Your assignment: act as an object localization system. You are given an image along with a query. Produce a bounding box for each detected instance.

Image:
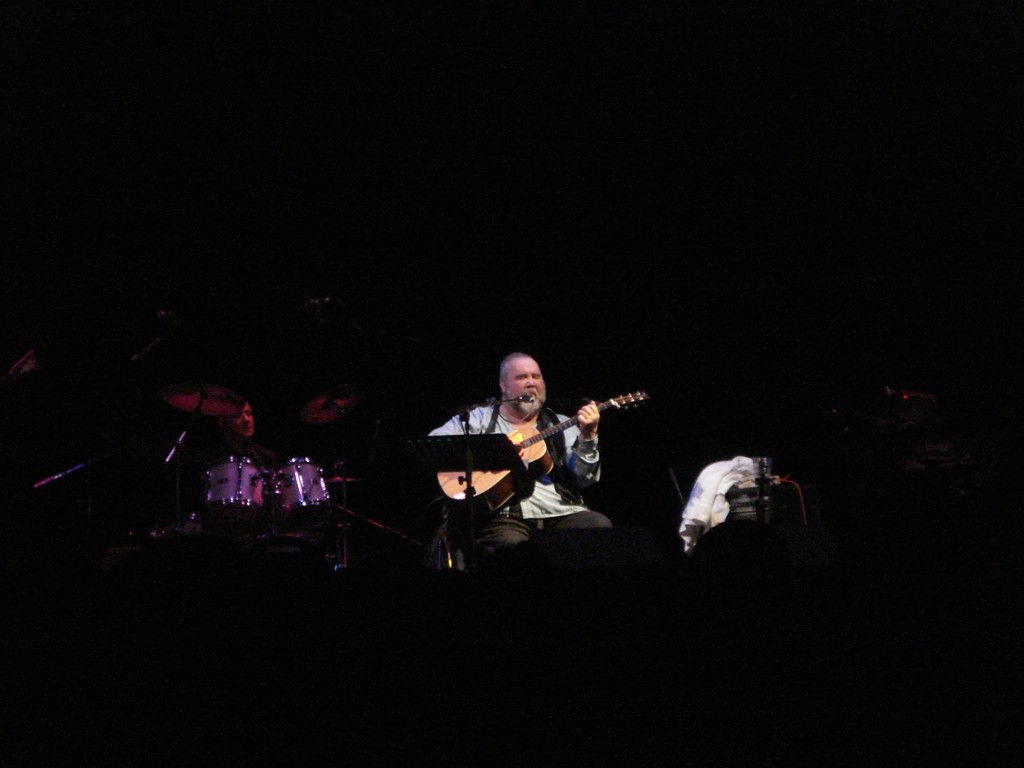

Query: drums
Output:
[180,515,204,536]
[196,453,262,531]
[266,457,332,526]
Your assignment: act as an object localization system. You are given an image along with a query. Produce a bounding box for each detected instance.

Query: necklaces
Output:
[498,405,531,423]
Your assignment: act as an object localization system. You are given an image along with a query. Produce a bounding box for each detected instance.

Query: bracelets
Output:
[572,435,599,454]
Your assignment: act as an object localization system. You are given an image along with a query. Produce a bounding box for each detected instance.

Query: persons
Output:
[677,454,774,552]
[427,353,610,544]
[200,400,276,469]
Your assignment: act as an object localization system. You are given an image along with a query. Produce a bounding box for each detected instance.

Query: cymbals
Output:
[300,382,357,425]
[324,475,362,485]
[162,384,245,418]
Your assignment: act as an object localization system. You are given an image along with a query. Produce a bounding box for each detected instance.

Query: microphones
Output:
[521,392,535,403]
[753,457,771,524]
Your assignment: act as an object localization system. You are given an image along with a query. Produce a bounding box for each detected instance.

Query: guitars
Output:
[437,390,652,515]
